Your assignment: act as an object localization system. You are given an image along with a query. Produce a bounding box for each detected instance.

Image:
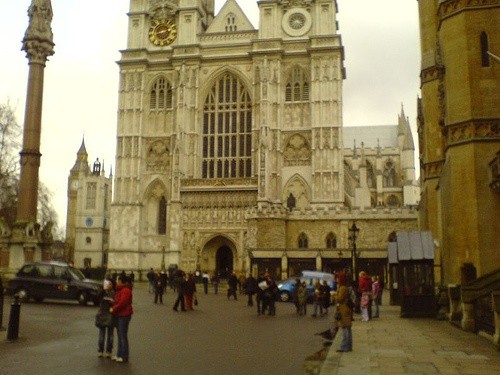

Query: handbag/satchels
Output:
[95,313,114,327]
[335,311,341,321]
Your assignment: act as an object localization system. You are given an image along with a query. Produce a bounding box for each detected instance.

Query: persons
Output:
[330,268,354,352]
[201,271,211,294]
[227,272,277,317]
[107,268,134,281]
[146,267,169,304]
[97,275,134,362]
[137,270,142,281]
[172,264,197,312]
[211,272,219,294]
[358,271,383,320]
[292,279,331,318]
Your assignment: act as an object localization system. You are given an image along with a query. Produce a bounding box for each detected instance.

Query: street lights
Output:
[348,221,361,284]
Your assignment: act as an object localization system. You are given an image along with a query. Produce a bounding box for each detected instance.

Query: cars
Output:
[3,262,103,307]
[275,270,340,303]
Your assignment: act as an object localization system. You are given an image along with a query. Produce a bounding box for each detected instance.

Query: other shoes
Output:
[105,352,111,358]
[115,357,124,362]
[99,352,104,357]
[173,308,178,311]
[111,355,116,360]
[336,348,350,352]
